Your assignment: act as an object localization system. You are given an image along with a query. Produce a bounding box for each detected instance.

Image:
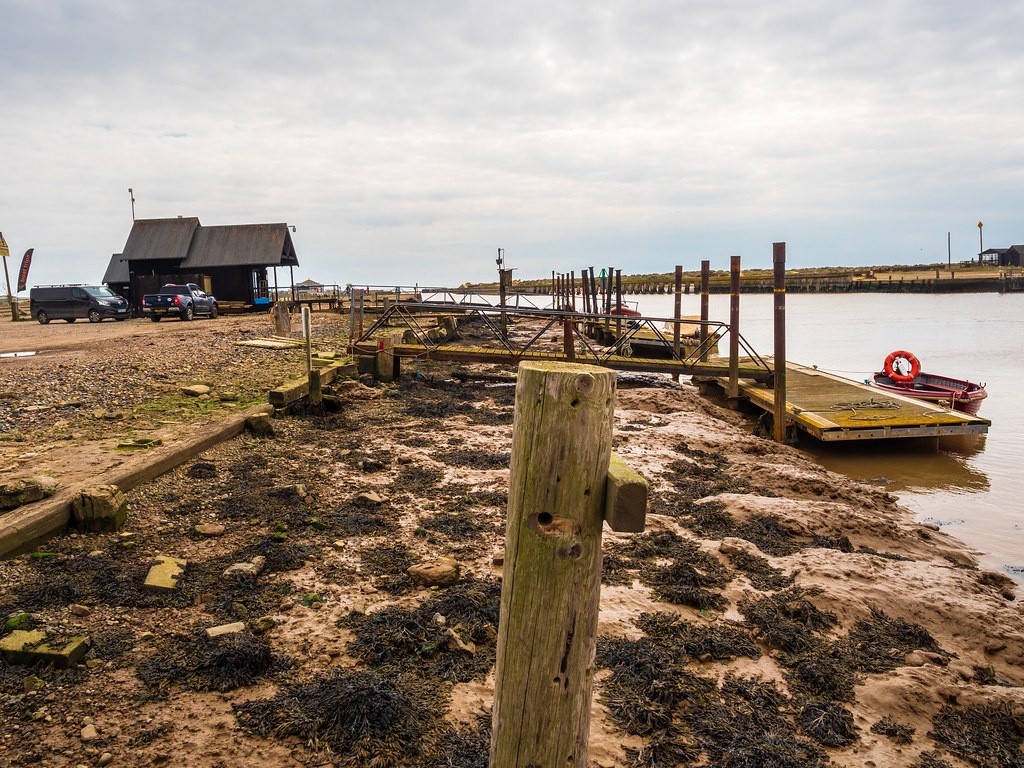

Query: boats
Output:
[601,301,641,320]
[663,313,720,349]
[865,368,988,417]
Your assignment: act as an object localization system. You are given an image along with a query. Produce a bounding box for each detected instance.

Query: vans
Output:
[30,282,130,323]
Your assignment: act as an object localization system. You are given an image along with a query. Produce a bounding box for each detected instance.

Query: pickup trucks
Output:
[143,283,219,322]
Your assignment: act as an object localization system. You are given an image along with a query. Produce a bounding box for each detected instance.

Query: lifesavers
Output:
[884,350,921,383]
